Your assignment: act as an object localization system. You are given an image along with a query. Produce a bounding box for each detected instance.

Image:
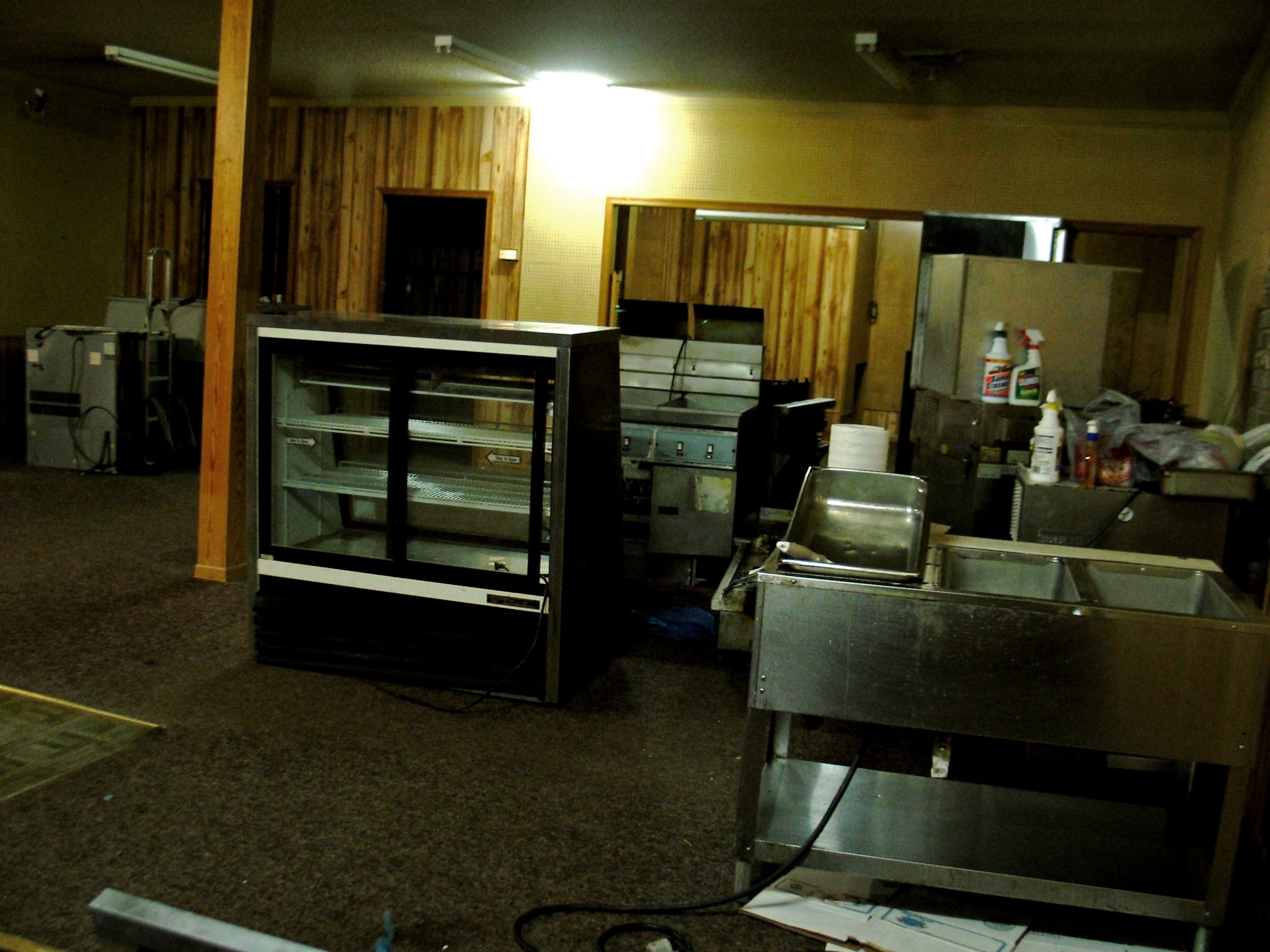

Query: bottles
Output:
[1031,389,1064,484]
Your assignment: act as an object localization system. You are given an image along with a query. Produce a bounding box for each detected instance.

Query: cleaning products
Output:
[980,320,1015,403]
[1028,386,1065,484]
[1007,327,1045,407]
[1081,418,1102,489]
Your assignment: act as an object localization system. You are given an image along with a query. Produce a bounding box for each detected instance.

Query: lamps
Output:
[434,35,531,85]
[854,33,913,92]
[105,45,218,86]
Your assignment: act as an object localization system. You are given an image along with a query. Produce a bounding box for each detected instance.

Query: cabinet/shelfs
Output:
[242,309,622,708]
